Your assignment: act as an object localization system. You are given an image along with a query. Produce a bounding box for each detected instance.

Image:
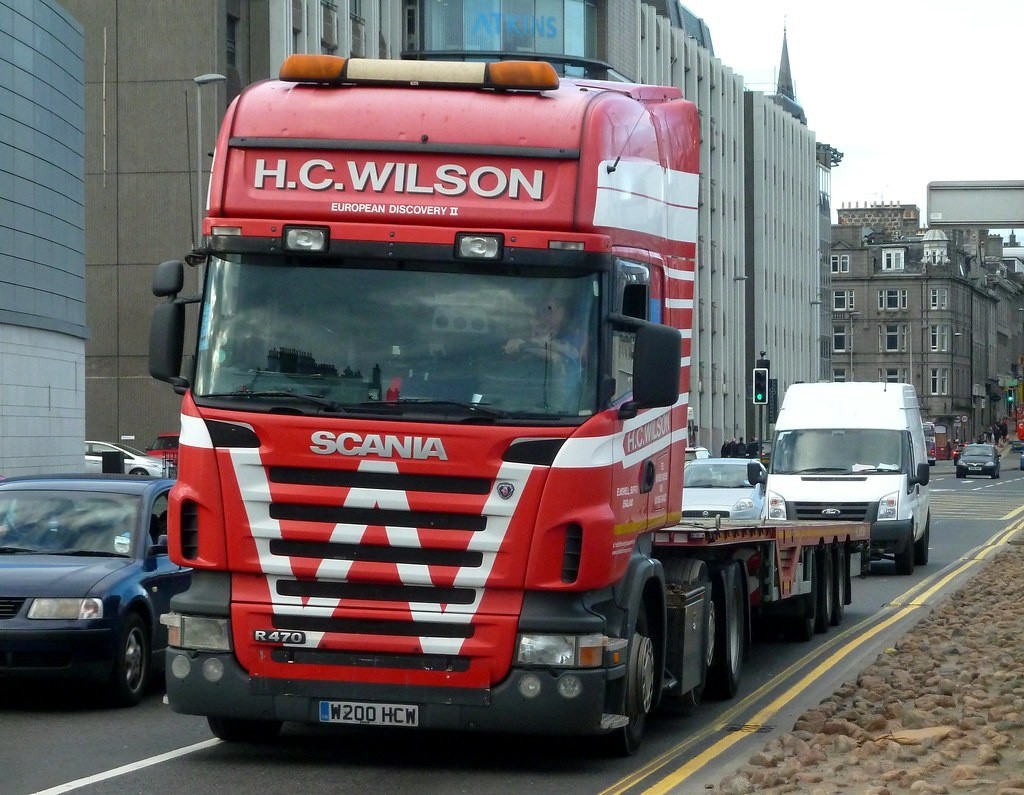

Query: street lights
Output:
[186,68,225,293]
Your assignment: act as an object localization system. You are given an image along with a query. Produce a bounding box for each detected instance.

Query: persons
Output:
[978,417,1008,445]
[504,296,587,370]
[946,439,952,457]
[720,438,759,459]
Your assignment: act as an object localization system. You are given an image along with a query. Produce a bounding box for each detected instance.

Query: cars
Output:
[86,439,176,477]
[955,444,1002,479]
[0,474,195,702]
[1020,448,1024,471]
[682,456,768,520]
[145,430,180,466]
[953,442,970,466]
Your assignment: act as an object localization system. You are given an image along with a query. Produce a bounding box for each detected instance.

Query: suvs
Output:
[684,445,711,463]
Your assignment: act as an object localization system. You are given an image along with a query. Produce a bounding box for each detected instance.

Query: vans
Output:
[746,379,932,577]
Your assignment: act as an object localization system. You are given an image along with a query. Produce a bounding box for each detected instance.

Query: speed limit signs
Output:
[960,414,970,424]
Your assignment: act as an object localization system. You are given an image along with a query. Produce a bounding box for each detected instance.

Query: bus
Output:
[922,421,937,467]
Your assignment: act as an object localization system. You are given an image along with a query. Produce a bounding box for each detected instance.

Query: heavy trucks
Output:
[138,44,876,757]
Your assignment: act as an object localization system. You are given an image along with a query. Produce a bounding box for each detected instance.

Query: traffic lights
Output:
[750,367,769,409]
[1007,388,1015,408]
